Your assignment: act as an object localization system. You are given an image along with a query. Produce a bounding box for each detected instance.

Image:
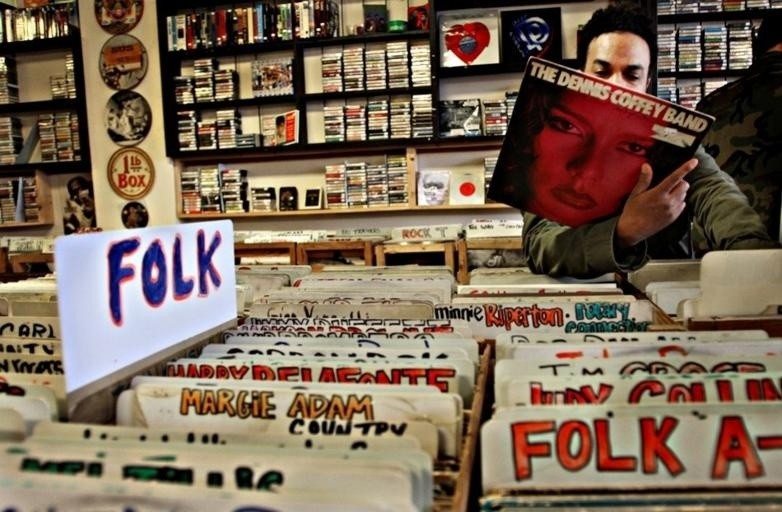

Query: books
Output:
[167,6,297,213]
[657,1,781,109]
[439,90,519,190]
[294,0,434,207]
[1,262,782,512]
[1,5,81,254]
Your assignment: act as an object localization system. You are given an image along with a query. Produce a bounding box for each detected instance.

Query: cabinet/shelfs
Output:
[1,1,777,284]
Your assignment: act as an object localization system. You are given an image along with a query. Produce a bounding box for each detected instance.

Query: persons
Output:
[692,14,782,257]
[522,6,773,279]
